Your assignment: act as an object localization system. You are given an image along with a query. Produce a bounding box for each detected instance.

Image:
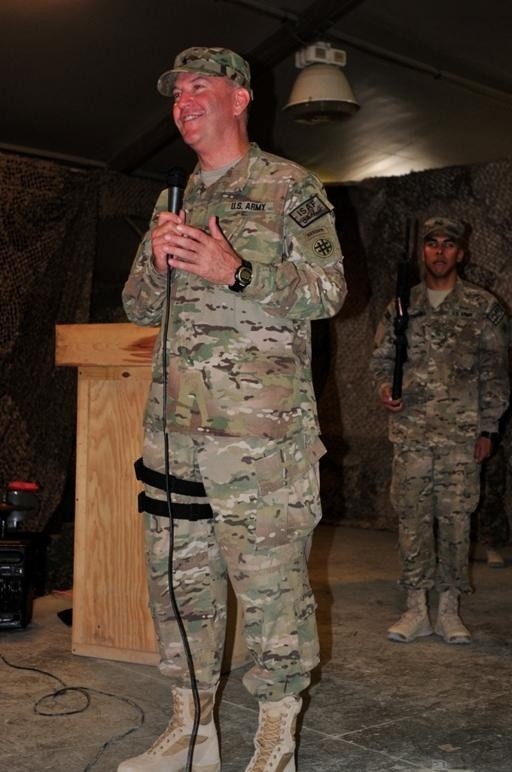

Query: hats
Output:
[422,215,467,242]
[156,46,252,98]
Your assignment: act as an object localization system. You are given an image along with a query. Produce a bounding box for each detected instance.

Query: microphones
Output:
[159,160,189,244]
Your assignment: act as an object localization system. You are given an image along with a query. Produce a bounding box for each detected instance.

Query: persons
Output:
[115,43,350,771]
[370,216,506,646]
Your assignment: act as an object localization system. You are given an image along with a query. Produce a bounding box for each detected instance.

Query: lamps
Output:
[279,44,364,127]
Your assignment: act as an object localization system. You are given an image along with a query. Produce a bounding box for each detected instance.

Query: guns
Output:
[392,219,421,401]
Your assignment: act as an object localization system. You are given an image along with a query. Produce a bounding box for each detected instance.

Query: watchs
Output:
[228,259,252,293]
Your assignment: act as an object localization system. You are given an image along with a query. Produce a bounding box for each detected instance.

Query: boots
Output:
[240,694,305,772]
[386,588,435,644]
[434,589,473,645]
[118,679,221,772]
